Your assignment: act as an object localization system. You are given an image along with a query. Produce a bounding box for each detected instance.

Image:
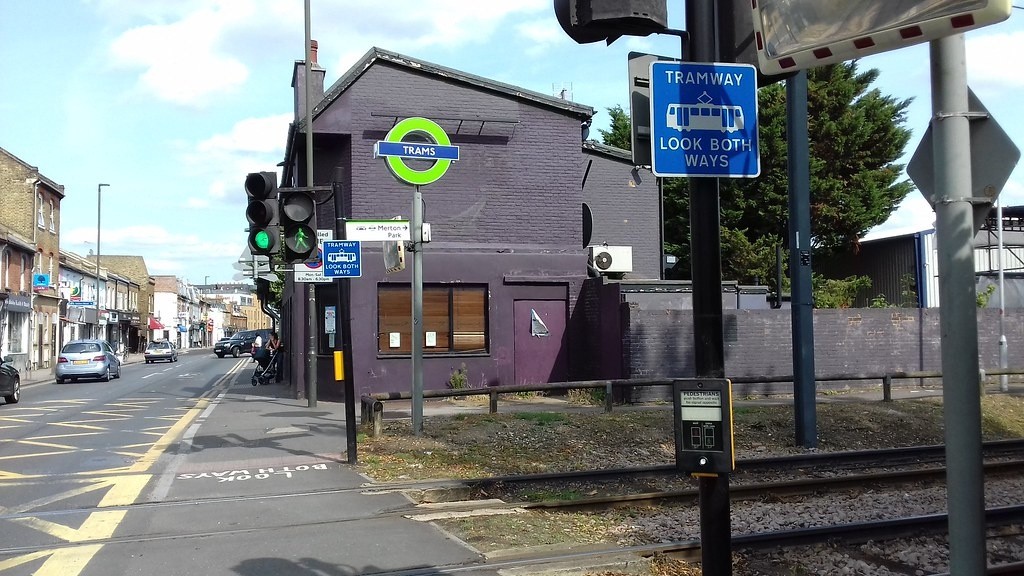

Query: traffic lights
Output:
[244,171,282,256]
[200,326,205,332]
[278,189,320,264]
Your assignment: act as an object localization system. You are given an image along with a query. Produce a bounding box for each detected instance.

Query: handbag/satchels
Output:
[251,344,256,355]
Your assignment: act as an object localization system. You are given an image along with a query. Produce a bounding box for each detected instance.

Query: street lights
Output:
[95,182,110,343]
[204,275,210,301]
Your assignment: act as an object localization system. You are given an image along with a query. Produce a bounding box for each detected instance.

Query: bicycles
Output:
[122,346,132,363]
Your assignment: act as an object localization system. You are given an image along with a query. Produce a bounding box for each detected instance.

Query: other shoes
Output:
[252,361,255,363]
[275,381,280,383]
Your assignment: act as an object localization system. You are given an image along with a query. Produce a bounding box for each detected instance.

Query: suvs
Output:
[214,328,273,358]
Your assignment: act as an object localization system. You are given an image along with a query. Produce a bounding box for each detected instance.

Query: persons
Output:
[252,332,262,363]
[266,332,284,379]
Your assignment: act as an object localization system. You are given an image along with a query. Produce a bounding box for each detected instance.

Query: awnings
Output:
[150,317,165,329]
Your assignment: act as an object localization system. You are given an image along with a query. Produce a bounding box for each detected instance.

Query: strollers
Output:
[251,347,279,387]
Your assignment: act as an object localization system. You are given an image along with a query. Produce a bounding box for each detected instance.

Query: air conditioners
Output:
[588,244,634,272]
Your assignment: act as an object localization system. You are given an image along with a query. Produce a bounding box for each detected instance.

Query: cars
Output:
[0,356,20,404]
[144,338,178,364]
[54,338,122,384]
